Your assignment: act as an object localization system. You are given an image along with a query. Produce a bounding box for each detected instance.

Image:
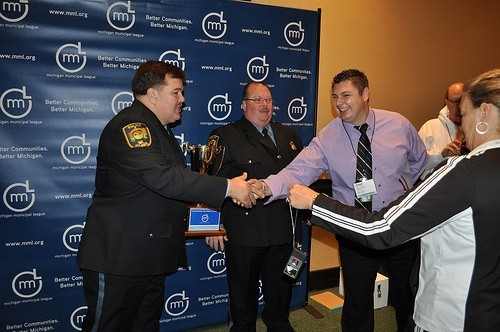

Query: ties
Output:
[261,128,276,148]
[353,123,372,213]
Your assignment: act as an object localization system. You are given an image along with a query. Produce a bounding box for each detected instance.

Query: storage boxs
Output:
[338,267,389,310]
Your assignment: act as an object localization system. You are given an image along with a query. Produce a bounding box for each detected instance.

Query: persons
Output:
[76,60,264,332]
[417,81,464,181]
[206,81,304,332]
[232,68,427,331]
[289,68,500,332]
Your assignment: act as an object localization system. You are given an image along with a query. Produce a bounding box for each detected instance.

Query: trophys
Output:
[179,134,226,237]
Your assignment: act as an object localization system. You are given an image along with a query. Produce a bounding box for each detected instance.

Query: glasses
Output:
[448,99,460,104]
[245,97,273,104]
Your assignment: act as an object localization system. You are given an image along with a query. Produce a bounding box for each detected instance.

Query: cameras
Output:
[282,247,308,280]
